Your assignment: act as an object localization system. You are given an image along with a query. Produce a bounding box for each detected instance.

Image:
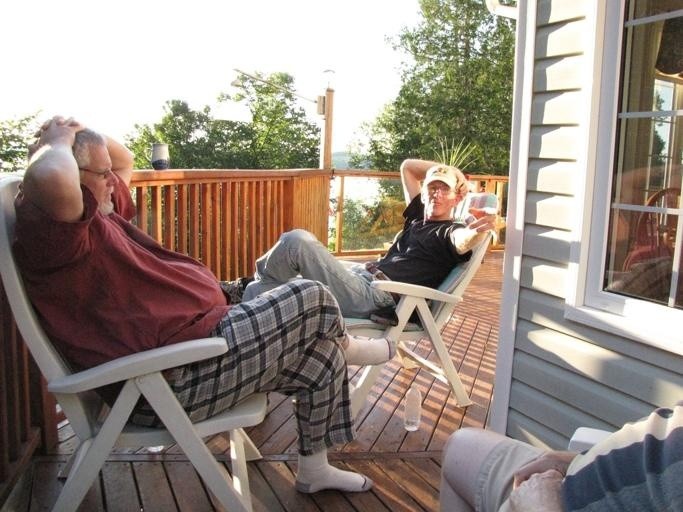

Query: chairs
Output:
[344,192,499,420]
[0,177,266,512]
[568,426,613,454]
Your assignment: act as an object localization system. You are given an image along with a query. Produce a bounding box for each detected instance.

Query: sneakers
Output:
[219,277,245,304]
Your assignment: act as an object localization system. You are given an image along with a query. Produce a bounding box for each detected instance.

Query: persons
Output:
[10,113,400,495]
[437,398,683,512]
[214,158,498,321]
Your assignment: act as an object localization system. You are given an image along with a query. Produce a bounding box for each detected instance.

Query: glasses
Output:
[427,184,455,196]
[78,165,112,181]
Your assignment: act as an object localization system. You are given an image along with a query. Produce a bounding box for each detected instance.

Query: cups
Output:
[145,143,171,170]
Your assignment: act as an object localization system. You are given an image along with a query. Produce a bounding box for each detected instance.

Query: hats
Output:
[423,165,458,191]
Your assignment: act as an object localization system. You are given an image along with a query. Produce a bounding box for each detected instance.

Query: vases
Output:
[152,144,169,168]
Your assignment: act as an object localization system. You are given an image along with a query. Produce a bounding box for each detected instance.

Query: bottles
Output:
[403,384,422,431]
